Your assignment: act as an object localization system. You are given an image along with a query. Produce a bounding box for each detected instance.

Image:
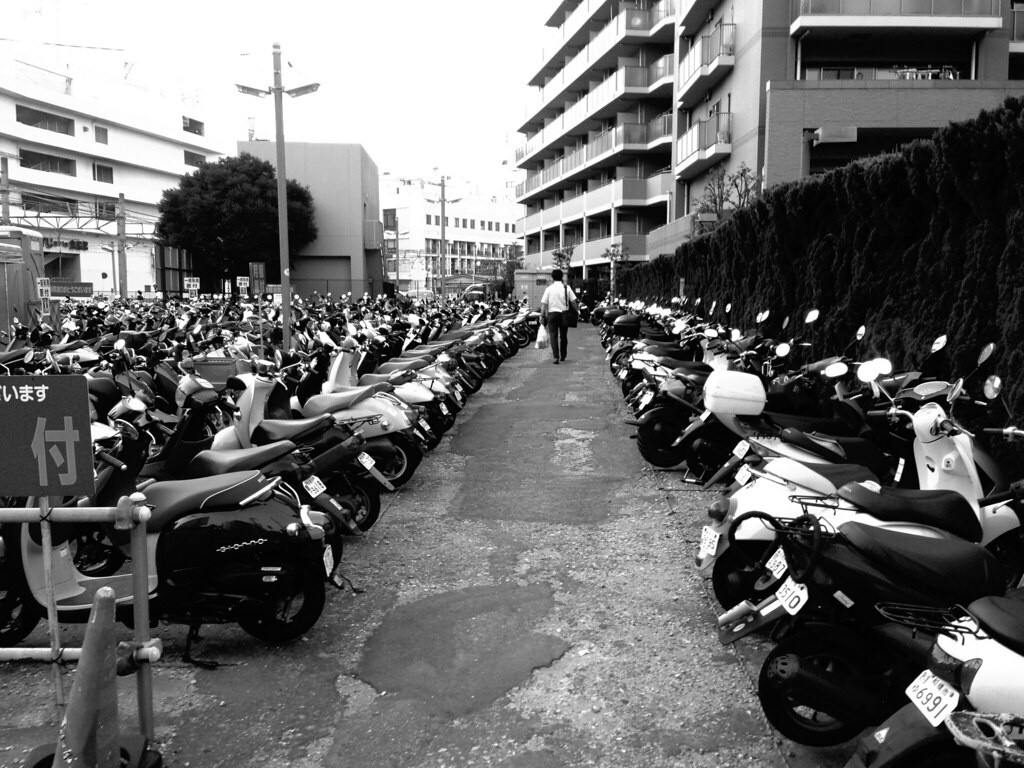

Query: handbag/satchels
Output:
[535,324,550,349]
[563,309,577,327]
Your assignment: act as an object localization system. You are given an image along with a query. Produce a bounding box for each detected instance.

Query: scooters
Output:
[574,286,1024,611]
[845,595,1024,768]
[0,417,333,660]
[716,513,1024,748]
[755,376,1024,641]
[0,292,548,588]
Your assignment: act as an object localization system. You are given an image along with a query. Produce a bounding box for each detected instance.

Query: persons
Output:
[539,269,579,364]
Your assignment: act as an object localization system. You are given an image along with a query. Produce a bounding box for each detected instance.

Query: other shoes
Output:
[553,358,559,364]
[561,355,566,361]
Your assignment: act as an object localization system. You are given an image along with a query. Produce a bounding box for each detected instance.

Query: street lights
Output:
[386,231,410,294]
[235,80,320,353]
[426,197,462,308]
